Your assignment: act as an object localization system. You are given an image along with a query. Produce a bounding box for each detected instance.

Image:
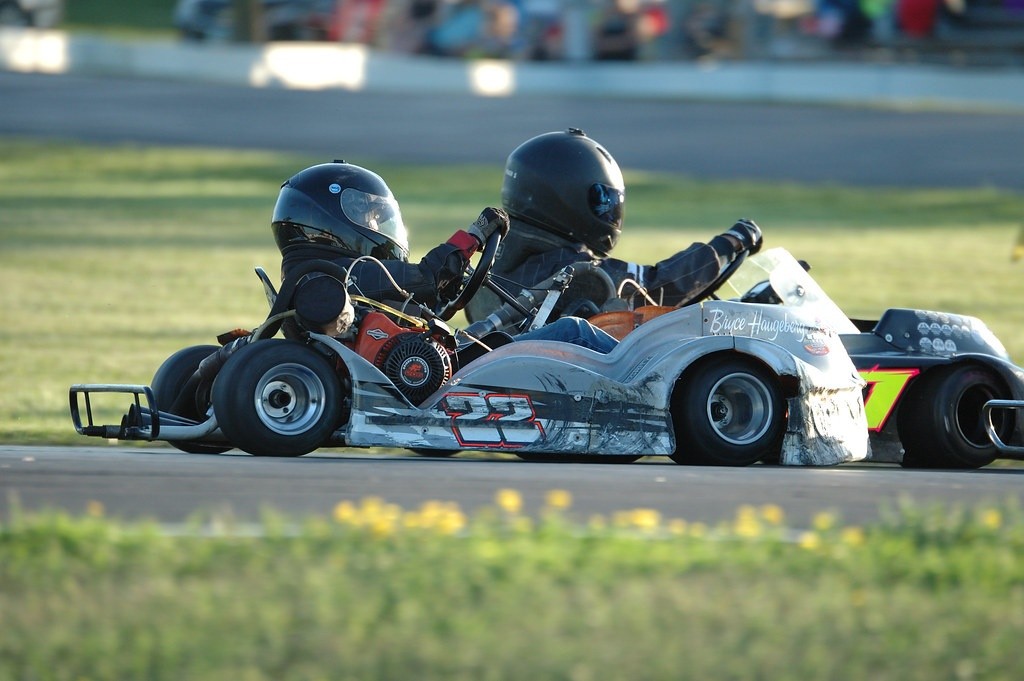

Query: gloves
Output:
[721,218,763,257]
[467,207,510,252]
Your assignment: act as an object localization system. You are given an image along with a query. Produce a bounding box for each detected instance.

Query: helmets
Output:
[271,160,410,281]
[502,127,625,255]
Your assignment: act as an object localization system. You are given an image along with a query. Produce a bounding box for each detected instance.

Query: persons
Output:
[272,159,621,357]
[464,126,764,335]
[0,0,1024,60]
